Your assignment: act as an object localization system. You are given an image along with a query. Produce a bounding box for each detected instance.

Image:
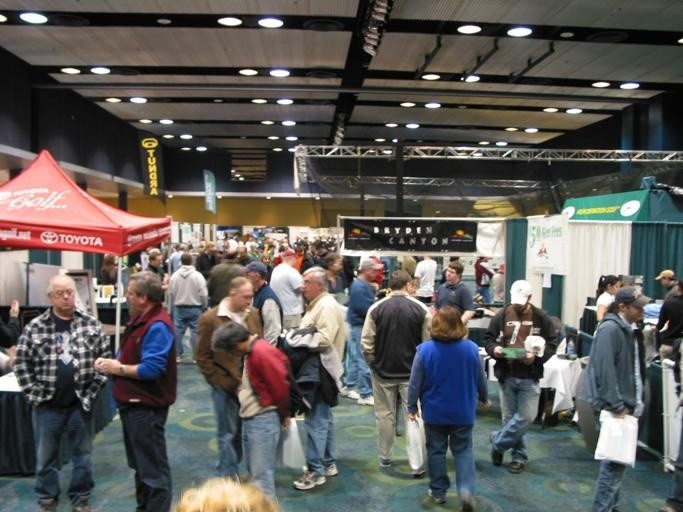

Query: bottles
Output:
[567,338,574,354]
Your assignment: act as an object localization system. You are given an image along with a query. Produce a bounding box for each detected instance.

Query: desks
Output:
[0,371,112,475]
[96,297,131,326]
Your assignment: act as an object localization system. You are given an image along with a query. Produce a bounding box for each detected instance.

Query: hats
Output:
[616,286,651,307]
[655,270,674,280]
[511,280,533,305]
[240,261,267,272]
[362,259,383,269]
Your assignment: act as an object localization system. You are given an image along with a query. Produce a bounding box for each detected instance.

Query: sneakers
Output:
[428,490,445,504]
[340,387,359,399]
[294,471,326,489]
[302,463,338,476]
[379,456,392,466]
[358,396,374,405]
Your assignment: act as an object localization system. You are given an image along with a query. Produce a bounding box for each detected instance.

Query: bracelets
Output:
[119,365,125,374]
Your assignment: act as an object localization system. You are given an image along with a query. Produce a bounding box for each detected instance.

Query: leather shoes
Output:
[509,461,524,473]
[490,431,503,466]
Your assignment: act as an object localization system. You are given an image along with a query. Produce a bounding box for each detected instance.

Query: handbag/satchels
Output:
[594,410,639,467]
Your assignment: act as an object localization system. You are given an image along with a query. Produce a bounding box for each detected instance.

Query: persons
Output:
[585,274,652,512]
[485,279,576,473]
[346,255,505,477]
[194,253,346,489]
[95,271,178,512]
[175,476,280,512]
[654,269,683,512]
[1,299,20,350]
[15,275,110,512]
[99,253,120,287]
[407,306,490,512]
[133,235,345,365]
